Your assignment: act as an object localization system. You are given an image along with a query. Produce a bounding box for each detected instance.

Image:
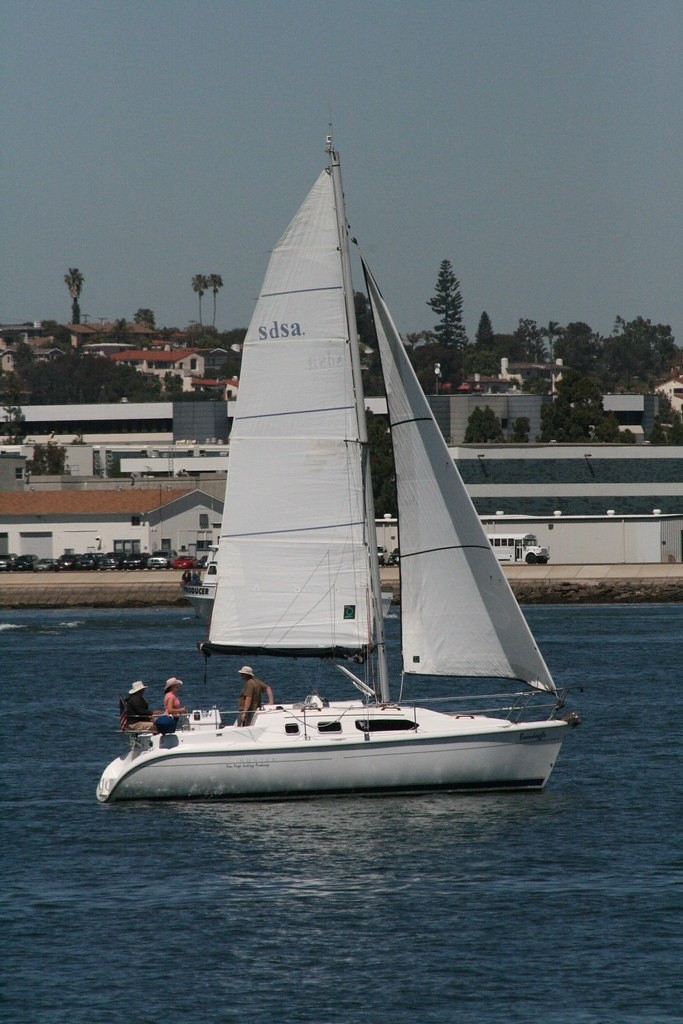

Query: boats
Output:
[179,546,218,620]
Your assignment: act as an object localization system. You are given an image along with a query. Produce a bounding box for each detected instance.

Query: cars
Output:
[376,545,386,567]
[33,558,58,572]
[198,555,209,567]
[172,554,200,570]
[387,547,400,566]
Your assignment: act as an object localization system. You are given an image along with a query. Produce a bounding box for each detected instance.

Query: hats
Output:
[165,677,183,690]
[238,666,254,676]
[128,681,148,694]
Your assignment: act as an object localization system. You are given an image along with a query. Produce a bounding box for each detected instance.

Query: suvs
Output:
[0,553,16,571]
[52,554,82,572]
[13,554,39,571]
[123,552,151,571]
[76,552,105,571]
[147,549,178,570]
[98,552,127,571]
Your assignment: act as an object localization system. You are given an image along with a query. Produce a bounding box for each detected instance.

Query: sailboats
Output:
[96,129,582,802]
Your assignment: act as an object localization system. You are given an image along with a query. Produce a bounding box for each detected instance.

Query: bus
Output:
[483,533,551,564]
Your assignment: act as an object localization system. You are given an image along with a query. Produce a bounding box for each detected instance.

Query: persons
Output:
[236,666,274,727]
[127,680,162,735]
[163,677,187,732]
[181,569,203,585]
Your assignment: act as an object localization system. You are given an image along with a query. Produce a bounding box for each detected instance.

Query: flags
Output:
[119,693,127,732]
